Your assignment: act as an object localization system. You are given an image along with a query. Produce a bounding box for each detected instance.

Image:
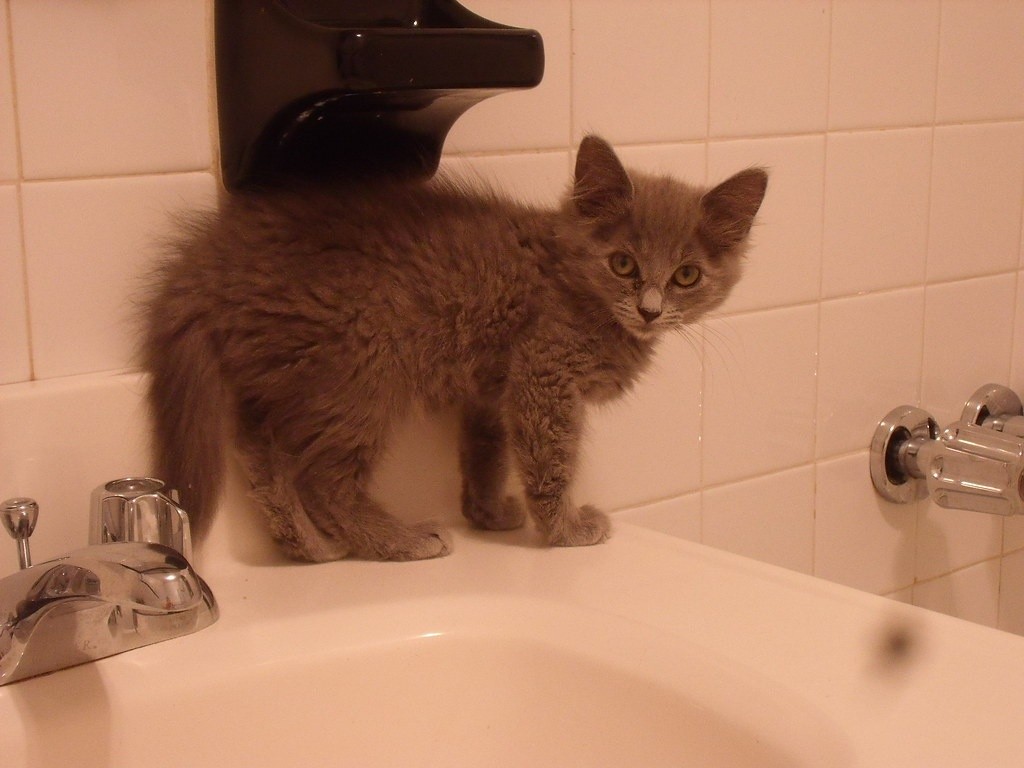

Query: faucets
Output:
[37,542,202,613]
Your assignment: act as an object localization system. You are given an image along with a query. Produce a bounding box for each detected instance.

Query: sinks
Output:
[1,518,1022,768]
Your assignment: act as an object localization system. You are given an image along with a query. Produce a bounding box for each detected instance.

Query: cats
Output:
[119,132,772,563]
